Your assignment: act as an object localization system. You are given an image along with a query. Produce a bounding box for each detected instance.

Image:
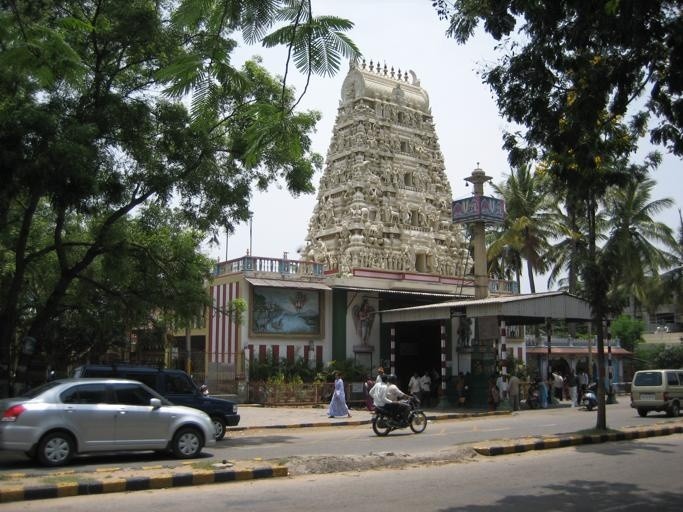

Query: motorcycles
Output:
[527,381,555,410]
[581,381,599,412]
[367,393,428,437]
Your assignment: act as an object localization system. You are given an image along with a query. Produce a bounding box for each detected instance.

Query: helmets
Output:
[389,374,398,383]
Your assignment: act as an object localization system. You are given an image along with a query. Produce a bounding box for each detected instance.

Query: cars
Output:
[1,376,219,470]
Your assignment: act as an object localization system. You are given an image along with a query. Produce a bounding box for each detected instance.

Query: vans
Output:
[63,358,241,441]
[628,368,683,418]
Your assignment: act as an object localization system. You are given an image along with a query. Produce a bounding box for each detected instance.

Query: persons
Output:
[359,303,369,346]
[463,318,472,347]
[326,372,351,418]
[365,367,589,430]
